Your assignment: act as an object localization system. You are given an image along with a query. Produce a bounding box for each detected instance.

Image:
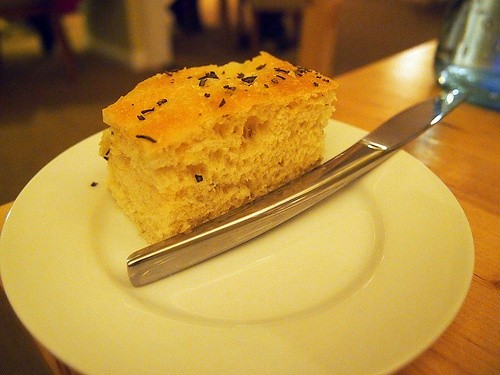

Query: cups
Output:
[433,1,500,110]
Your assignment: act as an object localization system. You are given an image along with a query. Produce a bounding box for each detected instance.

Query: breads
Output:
[102,50,337,245]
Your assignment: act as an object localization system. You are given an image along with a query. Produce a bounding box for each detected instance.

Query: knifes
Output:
[126,90,473,288]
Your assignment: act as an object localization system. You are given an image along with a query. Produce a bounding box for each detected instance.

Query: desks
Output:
[0,37,500,375]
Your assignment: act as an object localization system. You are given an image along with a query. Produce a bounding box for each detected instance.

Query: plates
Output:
[0,118,476,375]
